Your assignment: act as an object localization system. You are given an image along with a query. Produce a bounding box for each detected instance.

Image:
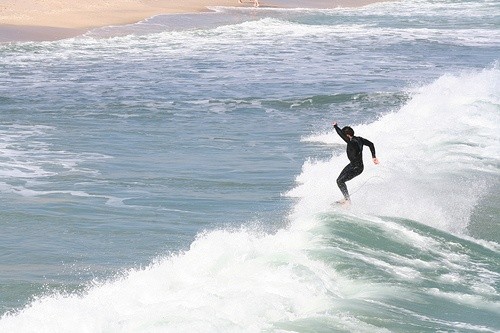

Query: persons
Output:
[332,120,380,205]
